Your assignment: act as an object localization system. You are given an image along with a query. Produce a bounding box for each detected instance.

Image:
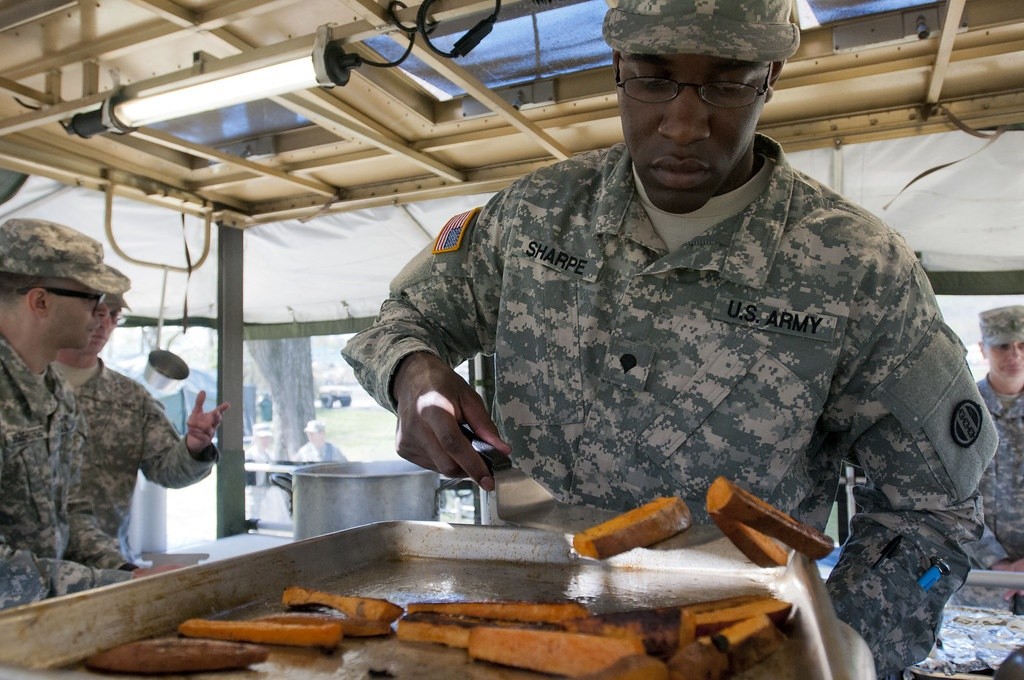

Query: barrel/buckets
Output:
[269,459,465,540]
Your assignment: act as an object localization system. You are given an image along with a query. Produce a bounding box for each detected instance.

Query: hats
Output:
[253,424,273,438]
[0,218,131,295]
[304,420,327,434]
[101,294,131,312]
[603,0,803,63]
[980,304,1024,348]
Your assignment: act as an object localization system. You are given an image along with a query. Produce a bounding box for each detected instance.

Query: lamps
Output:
[66,28,362,138]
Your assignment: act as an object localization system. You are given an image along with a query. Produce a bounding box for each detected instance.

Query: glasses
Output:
[16,284,107,316]
[615,49,775,109]
[97,305,127,326]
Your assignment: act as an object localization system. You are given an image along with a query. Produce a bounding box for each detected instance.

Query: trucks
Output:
[313,374,353,408]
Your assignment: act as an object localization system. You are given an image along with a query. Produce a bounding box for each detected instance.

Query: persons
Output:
[51,265,231,563]
[292,420,348,462]
[948,304,1024,611]
[0,217,182,608]
[245,422,274,519]
[339,0,999,680]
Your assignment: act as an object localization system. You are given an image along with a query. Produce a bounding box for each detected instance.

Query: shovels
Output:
[458,423,627,535]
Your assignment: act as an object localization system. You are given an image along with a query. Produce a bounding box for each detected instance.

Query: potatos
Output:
[83,473,837,680]
